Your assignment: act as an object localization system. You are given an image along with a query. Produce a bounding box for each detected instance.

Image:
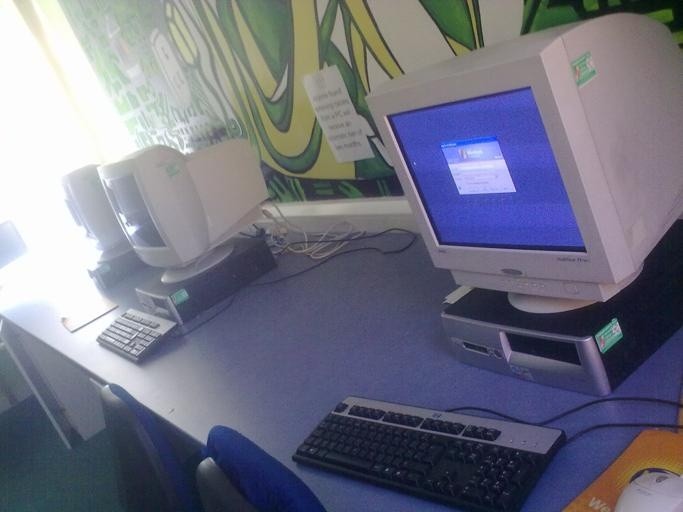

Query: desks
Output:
[0,233,683,511]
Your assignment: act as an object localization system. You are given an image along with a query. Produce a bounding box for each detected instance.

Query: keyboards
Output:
[96,308,178,362]
[292,395,567,512]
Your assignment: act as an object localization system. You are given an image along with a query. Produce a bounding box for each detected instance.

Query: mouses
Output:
[614,466,683,512]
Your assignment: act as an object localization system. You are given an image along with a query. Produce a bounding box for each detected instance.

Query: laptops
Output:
[365,12,682,315]
[96,137,270,283]
[52,164,136,261]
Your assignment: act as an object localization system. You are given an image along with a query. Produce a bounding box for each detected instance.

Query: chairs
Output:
[100,382,332,512]
